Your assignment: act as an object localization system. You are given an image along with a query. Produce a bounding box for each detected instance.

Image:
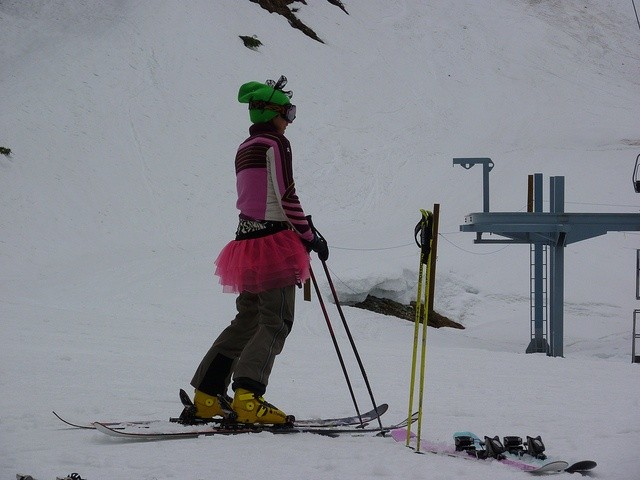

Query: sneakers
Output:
[230,388,287,424]
[194,388,234,419]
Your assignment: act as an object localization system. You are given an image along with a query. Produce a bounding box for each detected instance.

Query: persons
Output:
[190,75,329,425]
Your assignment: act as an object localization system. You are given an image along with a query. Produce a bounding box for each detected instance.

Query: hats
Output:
[238,81,290,124]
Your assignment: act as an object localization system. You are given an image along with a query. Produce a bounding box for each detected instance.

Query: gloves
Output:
[309,234,329,262]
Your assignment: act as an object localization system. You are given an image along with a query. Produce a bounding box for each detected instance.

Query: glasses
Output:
[249,99,296,123]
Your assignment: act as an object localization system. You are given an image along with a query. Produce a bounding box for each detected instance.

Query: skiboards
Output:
[390,428,596,473]
[52,404,419,437]
